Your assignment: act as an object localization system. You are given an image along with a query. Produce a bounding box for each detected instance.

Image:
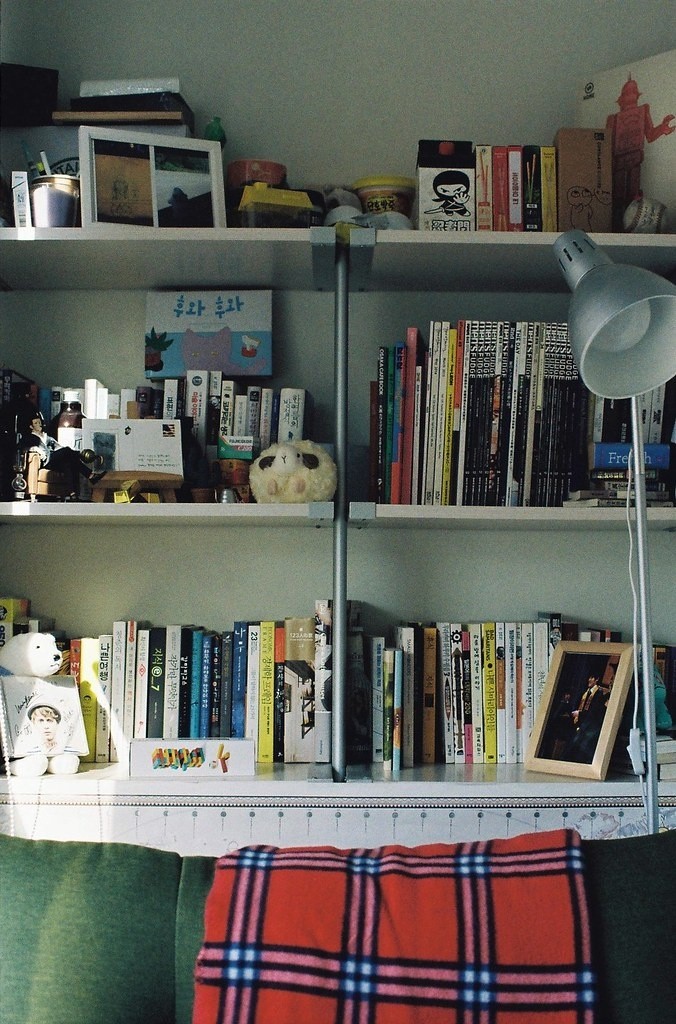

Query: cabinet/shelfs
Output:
[0,232,676,799]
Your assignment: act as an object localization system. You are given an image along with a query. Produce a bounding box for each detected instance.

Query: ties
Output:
[582,691,592,710]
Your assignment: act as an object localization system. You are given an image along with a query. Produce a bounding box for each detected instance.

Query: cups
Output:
[352,173,418,219]
[30,174,79,228]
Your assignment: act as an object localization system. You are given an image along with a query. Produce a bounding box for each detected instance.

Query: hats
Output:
[28,702,60,724]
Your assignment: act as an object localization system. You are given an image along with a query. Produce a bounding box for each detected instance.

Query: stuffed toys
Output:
[246,439,338,503]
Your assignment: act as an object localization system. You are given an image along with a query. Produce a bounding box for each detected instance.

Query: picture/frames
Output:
[521,639,639,783]
[78,124,227,229]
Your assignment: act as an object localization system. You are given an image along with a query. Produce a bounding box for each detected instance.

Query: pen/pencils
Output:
[19,139,40,178]
[35,161,47,176]
[40,149,52,177]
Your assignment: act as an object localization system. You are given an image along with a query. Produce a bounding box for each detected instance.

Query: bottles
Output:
[48,389,91,500]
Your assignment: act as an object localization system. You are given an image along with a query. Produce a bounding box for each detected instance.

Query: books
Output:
[0,367,309,504]
[142,284,274,382]
[368,313,676,507]
[410,125,616,234]
[352,607,676,781]
[44,107,187,128]
[0,595,333,775]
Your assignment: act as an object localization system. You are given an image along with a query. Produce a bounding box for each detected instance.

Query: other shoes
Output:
[70,494,83,503]
[90,471,108,485]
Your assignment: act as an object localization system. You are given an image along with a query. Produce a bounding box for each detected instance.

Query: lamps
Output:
[549,233,676,837]
